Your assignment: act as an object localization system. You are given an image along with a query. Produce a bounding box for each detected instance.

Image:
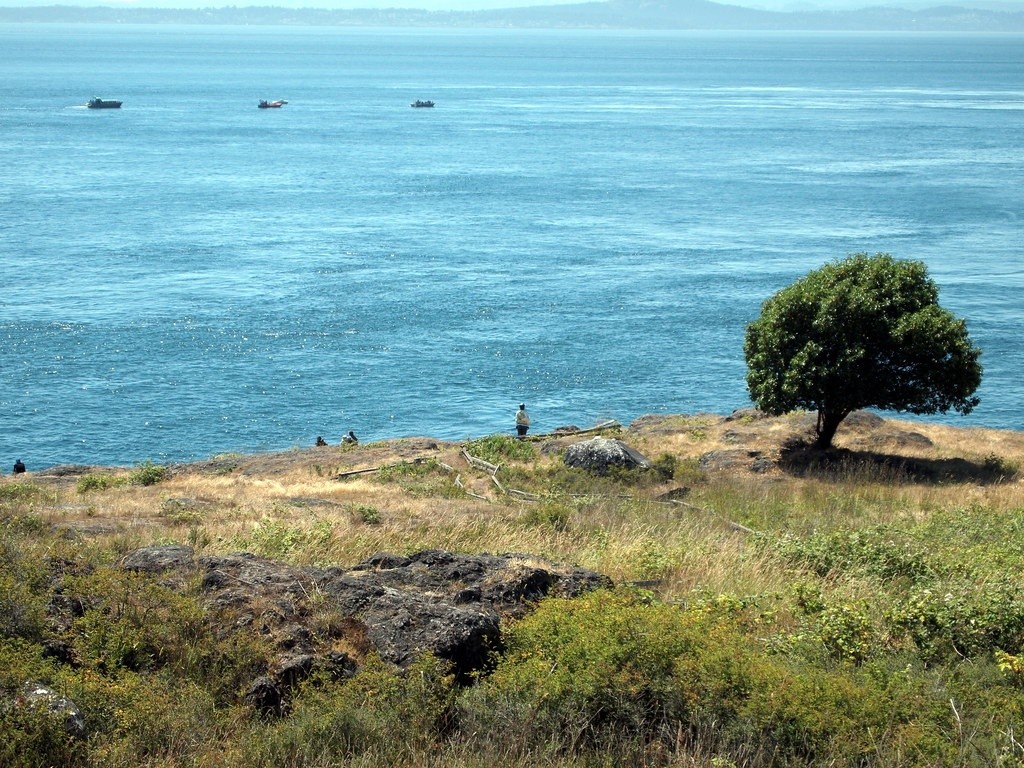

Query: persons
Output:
[341,435,348,446]
[316,436,327,446]
[347,431,358,446]
[515,403,529,436]
[14,458,26,474]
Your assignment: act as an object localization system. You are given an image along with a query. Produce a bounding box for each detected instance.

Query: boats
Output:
[410,99,435,107]
[258,98,282,108]
[85,96,123,108]
[273,99,288,104]
